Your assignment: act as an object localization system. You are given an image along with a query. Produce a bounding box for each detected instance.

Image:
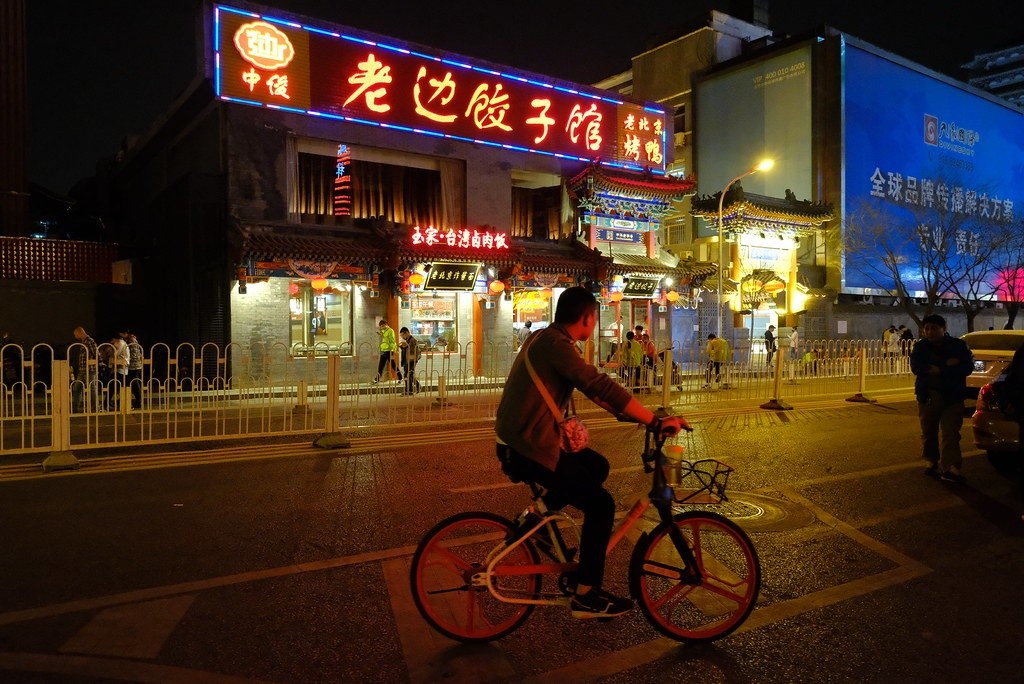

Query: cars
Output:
[971,364,1024,482]
[956,329,1024,400]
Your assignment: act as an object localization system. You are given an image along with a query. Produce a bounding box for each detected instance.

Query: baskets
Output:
[666,459,734,504]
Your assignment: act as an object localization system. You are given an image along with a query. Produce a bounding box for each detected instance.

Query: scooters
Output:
[625,346,683,393]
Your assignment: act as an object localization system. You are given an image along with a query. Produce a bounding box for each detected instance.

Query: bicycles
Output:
[409,406,762,646]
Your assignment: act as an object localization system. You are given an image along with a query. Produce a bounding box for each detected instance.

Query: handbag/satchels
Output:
[558,415,588,453]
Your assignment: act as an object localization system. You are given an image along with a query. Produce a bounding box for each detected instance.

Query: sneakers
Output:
[571,588,633,618]
[513,510,553,546]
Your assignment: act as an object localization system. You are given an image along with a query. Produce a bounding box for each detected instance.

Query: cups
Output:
[665,445,683,487]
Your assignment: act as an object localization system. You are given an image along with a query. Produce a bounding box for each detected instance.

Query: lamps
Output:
[422,263,432,272]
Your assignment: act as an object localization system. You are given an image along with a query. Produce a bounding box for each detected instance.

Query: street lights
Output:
[717,158,776,338]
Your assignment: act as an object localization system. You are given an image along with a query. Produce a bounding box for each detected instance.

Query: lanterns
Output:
[666,290,679,304]
[310,278,328,293]
[408,273,424,288]
[611,292,622,302]
[743,278,784,297]
[490,280,504,292]
[513,288,552,311]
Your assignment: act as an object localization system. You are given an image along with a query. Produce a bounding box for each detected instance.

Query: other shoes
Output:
[941,471,955,482]
[401,392,413,396]
[924,462,938,475]
[398,379,403,383]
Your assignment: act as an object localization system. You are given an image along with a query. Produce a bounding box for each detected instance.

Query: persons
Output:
[702,334,728,388]
[438,336,446,346]
[319,311,325,329]
[989,327,994,330]
[882,324,913,365]
[998,343,1024,519]
[911,314,973,482]
[74,326,141,412]
[517,321,532,343]
[764,325,862,377]
[495,287,689,620]
[370,320,422,397]
[607,316,657,394]
